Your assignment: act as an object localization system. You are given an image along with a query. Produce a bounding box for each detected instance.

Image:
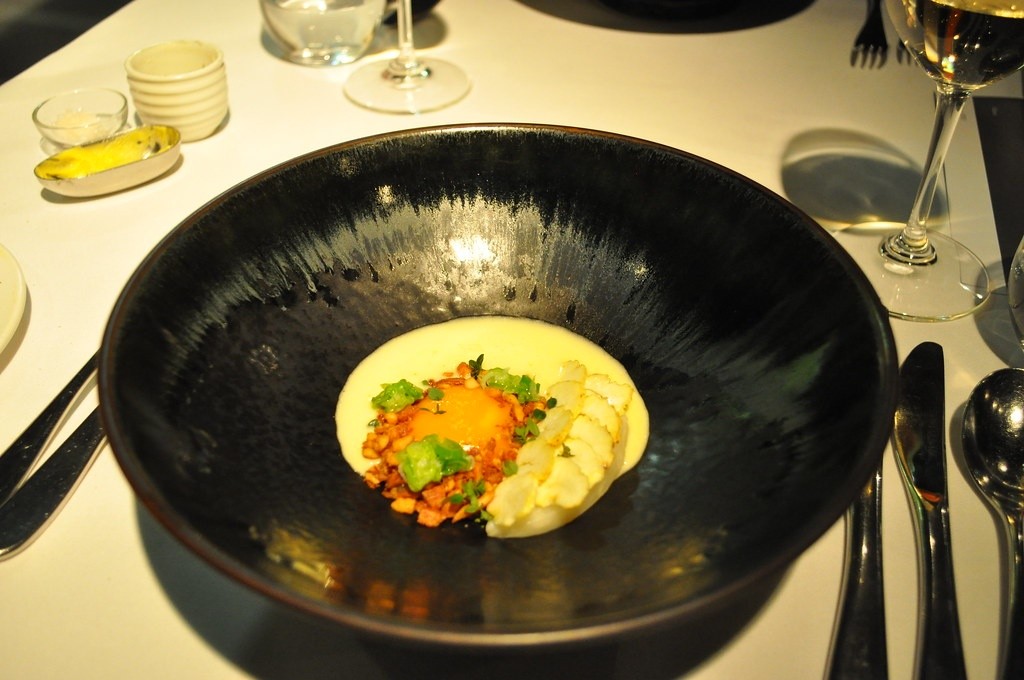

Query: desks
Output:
[0,0,1024,680]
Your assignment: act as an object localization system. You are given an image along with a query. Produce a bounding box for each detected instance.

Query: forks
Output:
[896,39,919,66]
[850,0,888,68]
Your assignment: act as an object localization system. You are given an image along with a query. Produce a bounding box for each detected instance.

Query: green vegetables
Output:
[368,351,559,523]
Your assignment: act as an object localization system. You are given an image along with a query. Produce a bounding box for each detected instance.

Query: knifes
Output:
[889,341,968,680]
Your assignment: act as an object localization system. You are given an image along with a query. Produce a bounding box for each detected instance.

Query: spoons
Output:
[960,368,1024,680]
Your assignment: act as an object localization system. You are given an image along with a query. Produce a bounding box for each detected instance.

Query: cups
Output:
[124,40,229,142]
[259,0,386,66]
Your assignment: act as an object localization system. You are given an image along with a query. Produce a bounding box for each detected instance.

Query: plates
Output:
[0,245,27,353]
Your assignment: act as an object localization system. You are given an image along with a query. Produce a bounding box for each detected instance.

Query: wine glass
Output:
[833,0,1024,324]
[342,0,471,115]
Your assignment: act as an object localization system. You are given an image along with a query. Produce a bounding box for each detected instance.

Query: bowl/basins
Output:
[31,87,128,151]
[34,124,183,198]
[96,122,902,654]
